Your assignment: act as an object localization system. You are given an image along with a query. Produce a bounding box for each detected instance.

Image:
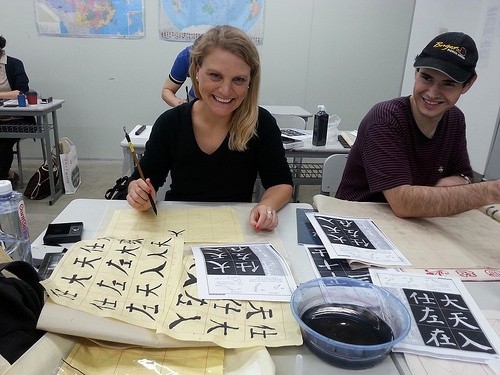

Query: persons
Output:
[0,36,38,179]
[127,24,295,233]
[334,32,500,218]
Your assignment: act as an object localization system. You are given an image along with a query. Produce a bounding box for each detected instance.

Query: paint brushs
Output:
[123,126,158,215]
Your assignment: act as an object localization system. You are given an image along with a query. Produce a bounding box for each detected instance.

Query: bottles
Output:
[0,179,34,268]
[312,104,329,146]
[18,92,26,107]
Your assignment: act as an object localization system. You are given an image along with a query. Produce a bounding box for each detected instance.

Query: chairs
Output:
[14,90,46,189]
[322,154,351,201]
[276,116,306,202]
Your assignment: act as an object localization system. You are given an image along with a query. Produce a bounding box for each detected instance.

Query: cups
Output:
[26,90,38,105]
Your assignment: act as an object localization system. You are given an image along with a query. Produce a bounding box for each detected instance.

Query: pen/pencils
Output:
[186,86,189,102]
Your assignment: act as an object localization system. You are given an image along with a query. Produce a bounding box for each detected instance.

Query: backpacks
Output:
[0,261,49,365]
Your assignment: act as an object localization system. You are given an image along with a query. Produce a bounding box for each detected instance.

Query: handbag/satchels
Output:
[24,137,80,200]
[105,176,131,200]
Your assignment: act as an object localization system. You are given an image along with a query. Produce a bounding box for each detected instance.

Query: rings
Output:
[268,211,273,214]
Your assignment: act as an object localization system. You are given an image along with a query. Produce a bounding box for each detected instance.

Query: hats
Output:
[413,32,478,83]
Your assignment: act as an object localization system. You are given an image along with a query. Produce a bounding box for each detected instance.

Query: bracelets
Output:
[460,173,470,182]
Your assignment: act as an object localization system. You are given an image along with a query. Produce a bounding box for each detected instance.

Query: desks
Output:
[121,126,358,203]
[0,99,64,206]
[30,199,500,375]
[260,105,313,130]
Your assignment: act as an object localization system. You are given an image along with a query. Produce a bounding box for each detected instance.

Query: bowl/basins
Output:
[290,276,411,371]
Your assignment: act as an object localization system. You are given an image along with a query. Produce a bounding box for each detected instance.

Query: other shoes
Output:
[6,172,19,190]
